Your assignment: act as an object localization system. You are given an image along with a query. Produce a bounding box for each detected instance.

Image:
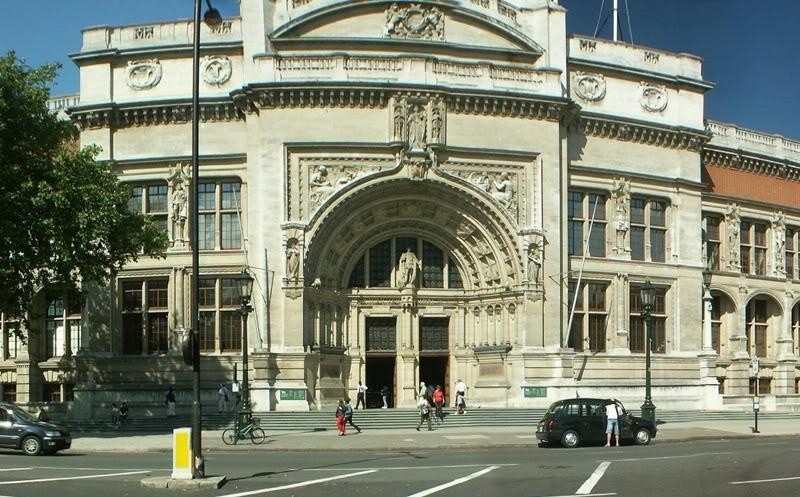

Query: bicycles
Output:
[222,411,266,446]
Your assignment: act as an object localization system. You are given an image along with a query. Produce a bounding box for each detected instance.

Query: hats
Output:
[436,385,441,389]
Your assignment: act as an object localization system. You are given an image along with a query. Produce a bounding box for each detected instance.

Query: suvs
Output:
[534,396,657,447]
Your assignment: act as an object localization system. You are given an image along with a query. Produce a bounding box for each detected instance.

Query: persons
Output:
[335,399,347,435]
[416,378,468,432]
[400,248,419,284]
[605,399,620,447]
[286,243,299,279]
[118,401,128,428]
[216,383,229,412]
[309,166,330,192]
[614,197,629,251]
[492,172,513,209]
[165,388,176,416]
[353,381,368,410]
[170,184,188,221]
[725,219,739,267]
[773,227,788,273]
[381,385,388,409]
[408,106,426,153]
[111,403,119,424]
[528,248,541,282]
[344,399,361,432]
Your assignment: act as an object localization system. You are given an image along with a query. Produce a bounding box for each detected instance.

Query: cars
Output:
[0,400,70,455]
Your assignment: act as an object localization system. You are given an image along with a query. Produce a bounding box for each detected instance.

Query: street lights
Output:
[640,277,656,422]
[186,0,220,480]
[698,268,718,386]
[237,262,256,437]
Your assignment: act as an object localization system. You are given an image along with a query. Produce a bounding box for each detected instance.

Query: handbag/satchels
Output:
[225,394,228,402]
